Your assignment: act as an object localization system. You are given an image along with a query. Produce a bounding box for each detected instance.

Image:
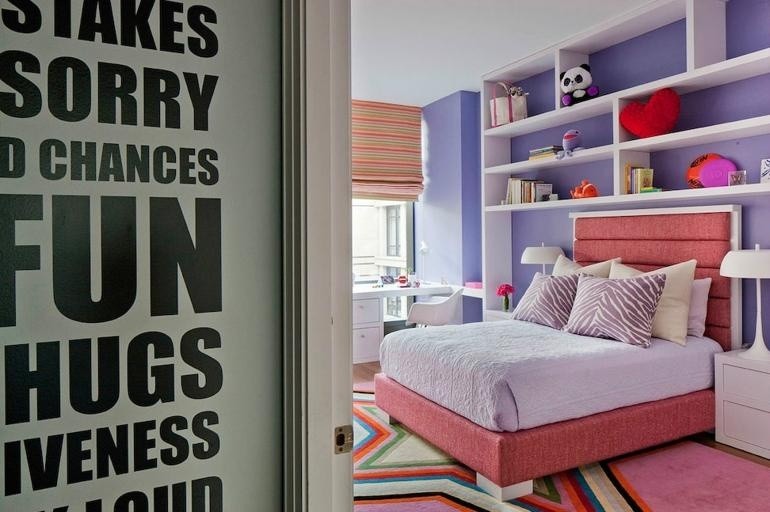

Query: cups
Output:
[408,271,416,284]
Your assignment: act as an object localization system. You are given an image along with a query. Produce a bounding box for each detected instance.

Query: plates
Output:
[686,152,722,189]
[701,158,737,189]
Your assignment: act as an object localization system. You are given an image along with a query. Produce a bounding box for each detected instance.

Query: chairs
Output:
[406,287,465,328]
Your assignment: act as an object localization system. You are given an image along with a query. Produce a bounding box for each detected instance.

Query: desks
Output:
[352,278,453,365]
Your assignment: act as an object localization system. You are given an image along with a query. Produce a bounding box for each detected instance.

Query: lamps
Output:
[519,240,566,276]
[719,243,770,361]
[417,239,431,285]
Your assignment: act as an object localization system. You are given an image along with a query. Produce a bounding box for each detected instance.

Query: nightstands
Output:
[713,348,770,461]
[486,308,514,321]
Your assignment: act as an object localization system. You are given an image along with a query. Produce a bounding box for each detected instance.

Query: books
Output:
[505,178,552,203]
[625,164,662,193]
[528,145,563,161]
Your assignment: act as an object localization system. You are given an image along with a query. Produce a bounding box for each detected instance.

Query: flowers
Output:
[495,284,515,309]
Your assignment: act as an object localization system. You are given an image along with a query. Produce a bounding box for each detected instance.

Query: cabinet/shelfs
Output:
[481,0,770,211]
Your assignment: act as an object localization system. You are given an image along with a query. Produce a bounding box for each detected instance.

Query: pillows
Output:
[551,254,622,278]
[512,272,580,332]
[687,276,713,340]
[608,259,698,347]
[559,272,668,349]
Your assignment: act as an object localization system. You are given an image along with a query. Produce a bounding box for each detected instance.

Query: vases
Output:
[502,295,511,312]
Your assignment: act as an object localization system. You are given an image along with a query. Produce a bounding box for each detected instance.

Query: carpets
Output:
[353,378,769,512]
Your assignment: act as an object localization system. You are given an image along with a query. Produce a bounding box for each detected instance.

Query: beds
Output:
[373,202,744,504]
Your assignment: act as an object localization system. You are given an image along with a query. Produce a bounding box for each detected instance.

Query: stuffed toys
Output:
[560,64,600,105]
[554,130,586,159]
[569,180,599,199]
